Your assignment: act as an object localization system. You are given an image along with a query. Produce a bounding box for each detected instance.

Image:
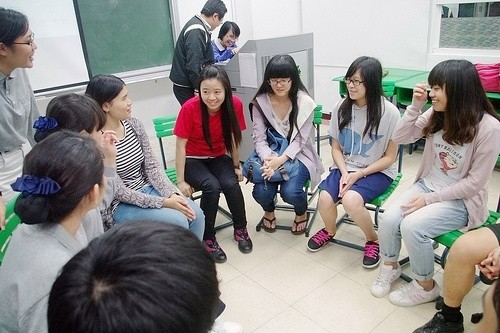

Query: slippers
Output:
[263,214,277,233]
[291,211,310,235]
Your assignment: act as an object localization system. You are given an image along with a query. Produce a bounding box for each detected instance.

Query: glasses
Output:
[5,33,35,46]
[268,78,291,85]
[226,34,239,41]
[343,76,364,85]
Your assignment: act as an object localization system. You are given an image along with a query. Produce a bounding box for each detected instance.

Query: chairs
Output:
[0,192,24,268]
[381,80,414,173]
[152,114,236,233]
[326,111,410,251]
[399,152,500,310]
[255,103,324,237]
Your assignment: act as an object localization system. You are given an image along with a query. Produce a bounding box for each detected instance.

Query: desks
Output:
[214,31,315,163]
[332,67,429,99]
[396,70,500,169]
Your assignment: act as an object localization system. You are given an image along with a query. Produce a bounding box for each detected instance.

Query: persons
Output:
[34,93,120,246]
[1,3,45,230]
[211,20,241,64]
[411,223,500,333]
[250,54,325,235]
[84,72,208,246]
[370,58,500,307]
[1,130,109,333]
[308,57,401,270]
[470,278,500,333]
[175,64,254,264]
[170,0,227,107]
[48,220,220,332]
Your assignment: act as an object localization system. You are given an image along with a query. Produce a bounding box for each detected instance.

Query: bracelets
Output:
[234,163,240,170]
[359,169,367,178]
[194,90,200,93]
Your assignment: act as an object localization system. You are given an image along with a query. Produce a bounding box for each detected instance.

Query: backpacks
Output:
[474,62,500,91]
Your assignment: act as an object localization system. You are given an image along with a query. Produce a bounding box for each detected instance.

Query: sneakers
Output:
[362,241,381,269]
[390,278,441,309]
[234,227,252,253]
[307,226,335,252]
[201,237,227,263]
[410,312,465,333]
[371,261,402,297]
